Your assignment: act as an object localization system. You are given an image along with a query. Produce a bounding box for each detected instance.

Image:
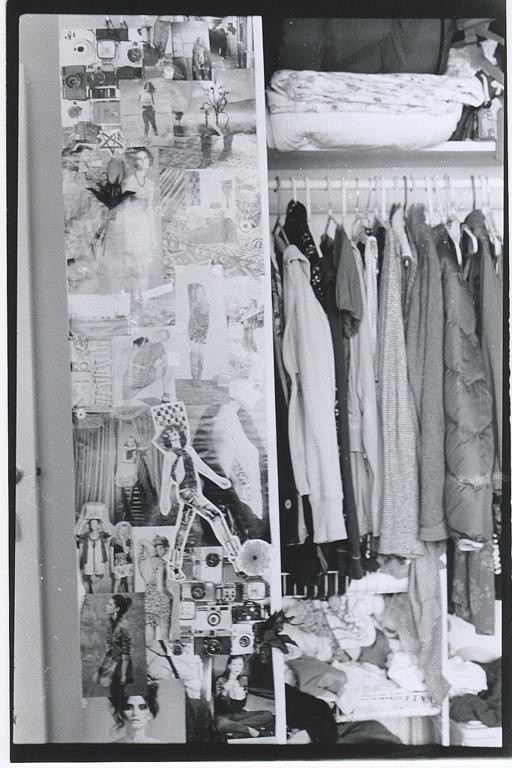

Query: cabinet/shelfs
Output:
[57,10,509,752]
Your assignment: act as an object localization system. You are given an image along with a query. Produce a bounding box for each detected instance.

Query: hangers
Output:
[267,169,510,281]
